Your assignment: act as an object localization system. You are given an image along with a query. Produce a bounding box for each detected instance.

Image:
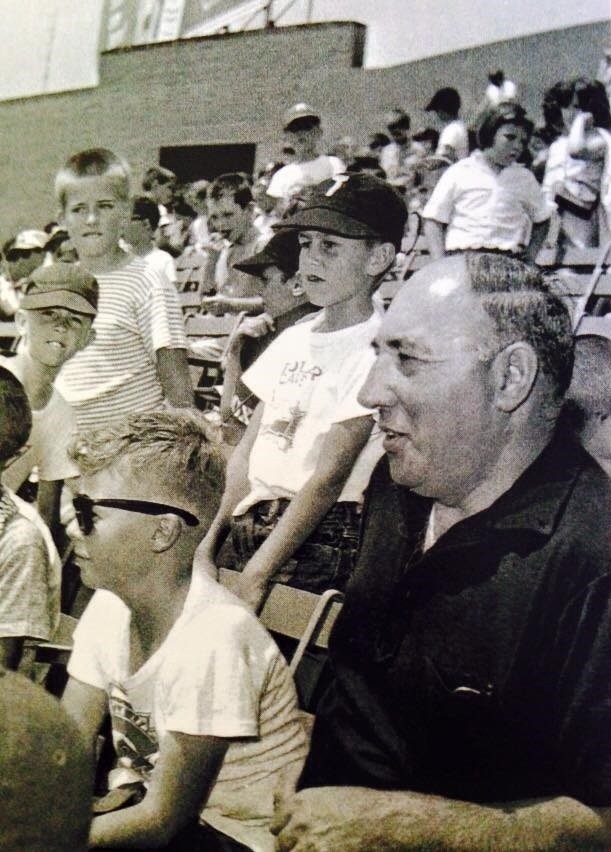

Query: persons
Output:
[59,410,310,852]
[0,261,99,534]
[0,365,63,689]
[194,172,409,615]
[0,670,93,852]
[0,47,611,475]
[266,247,611,851]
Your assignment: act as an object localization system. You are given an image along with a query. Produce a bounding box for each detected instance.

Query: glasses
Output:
[73,497,200,537]
[11,252,31,263]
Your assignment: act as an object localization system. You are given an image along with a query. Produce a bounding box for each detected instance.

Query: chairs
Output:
[213,568,347,692]
[172,256,249,409]
[379,232,611,337]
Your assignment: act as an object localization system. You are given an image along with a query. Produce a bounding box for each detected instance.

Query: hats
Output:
[270,173,408,240]
[21,264,100,318]
[233,228,300,275]
[281,103,321,131]
[426,87,461,111]
[9,230,50,249]
